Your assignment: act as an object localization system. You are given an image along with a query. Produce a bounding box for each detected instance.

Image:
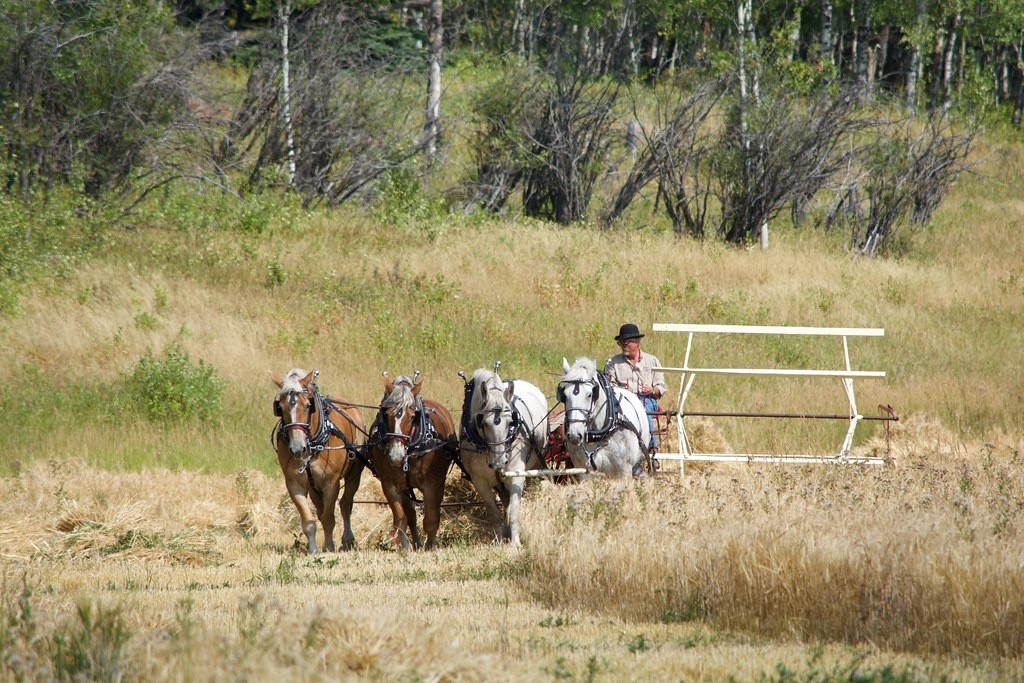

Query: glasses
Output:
[617,338,636,346]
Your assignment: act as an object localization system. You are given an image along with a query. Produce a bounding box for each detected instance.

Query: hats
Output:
[614,324,645,340]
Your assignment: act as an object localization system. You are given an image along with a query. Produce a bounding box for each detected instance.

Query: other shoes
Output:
[653,459,660,469]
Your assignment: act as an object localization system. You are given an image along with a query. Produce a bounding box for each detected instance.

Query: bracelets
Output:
[653,388,655,394]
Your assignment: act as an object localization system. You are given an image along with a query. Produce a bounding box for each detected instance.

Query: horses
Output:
[269,357,651,554]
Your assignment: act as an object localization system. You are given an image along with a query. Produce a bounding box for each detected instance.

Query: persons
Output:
[604,324,667,454]
